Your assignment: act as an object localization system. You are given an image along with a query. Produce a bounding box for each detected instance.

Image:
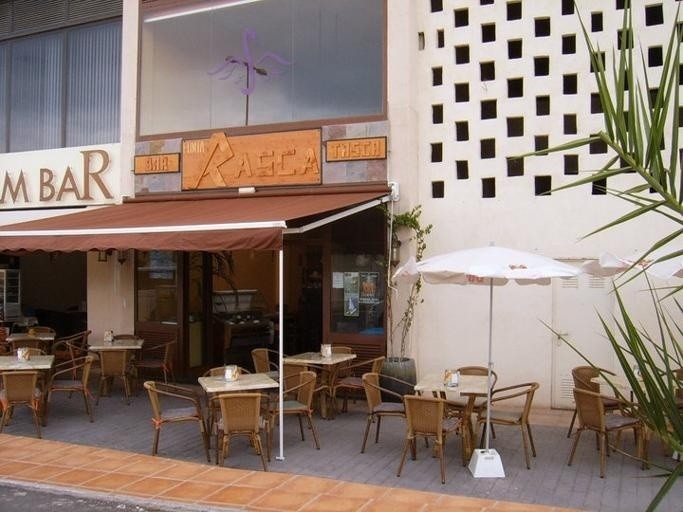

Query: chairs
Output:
[396,395,467,485]
[8,348,48,418]
[28,326,55,354]
[331,347,356,413]
[92,349,134,406]
[329,356,386,423]
[40,356,94,427]
[446,366,497,439]
[568,386,651,479]
[283,363,332,421]
[127,340,176,397]
[661,368,683,419]
[251,347,289,406]
[0,371,47,439]
[144,381,212,463]
[266,370,321,451]
[361,373,429,454]
[51,330,92,381]
[566,366,634,450]
[202,366,252,433]
[468,382,540,470]
[207,393,272,472]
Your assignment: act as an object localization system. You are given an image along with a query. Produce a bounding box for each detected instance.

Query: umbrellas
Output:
[392,246,582,479]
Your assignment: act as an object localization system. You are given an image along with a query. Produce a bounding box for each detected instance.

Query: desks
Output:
[89,338,144,397]
[0,354,54,415]
[283,352,357,419]
[6,333,55,351]
[197,373,280,449]
[414,372,493,461]
